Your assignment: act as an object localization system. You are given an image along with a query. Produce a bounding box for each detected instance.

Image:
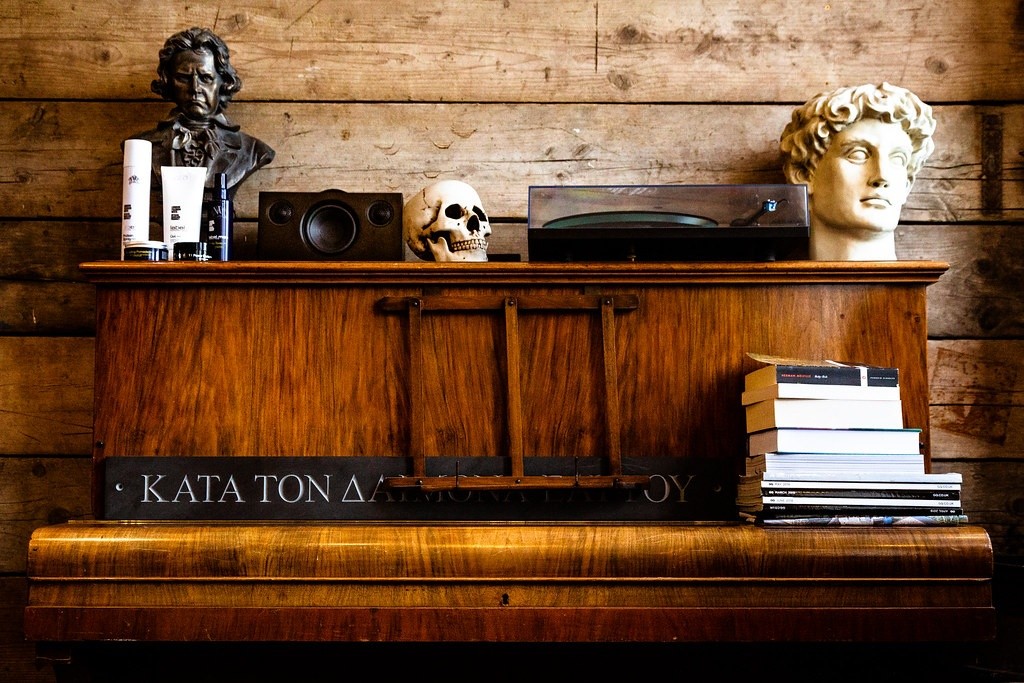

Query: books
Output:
[740,352,969,530]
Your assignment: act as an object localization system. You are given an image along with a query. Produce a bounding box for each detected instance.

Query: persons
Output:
[780,83,937,262]
[120,27,276,224]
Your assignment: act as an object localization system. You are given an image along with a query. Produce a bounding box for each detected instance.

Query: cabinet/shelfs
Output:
[21,259,999,672]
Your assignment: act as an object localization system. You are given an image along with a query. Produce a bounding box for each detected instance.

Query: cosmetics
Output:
[121,138,233,261]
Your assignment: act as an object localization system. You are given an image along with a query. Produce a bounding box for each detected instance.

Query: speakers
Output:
[256,189,406,264]
